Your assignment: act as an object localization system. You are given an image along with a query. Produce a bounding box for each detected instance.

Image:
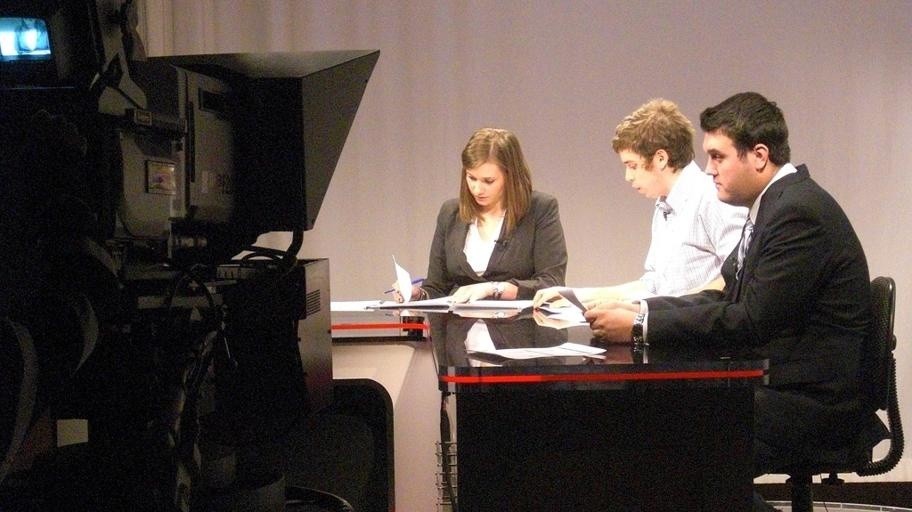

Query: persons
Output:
[532,309,591,331]
[590,337,760,366]
[391,308,567,370]
[530,96,749,318]
[582,91,890,511]
[390,126,569,307]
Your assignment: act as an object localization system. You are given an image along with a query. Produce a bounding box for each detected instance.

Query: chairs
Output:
[754,276,904,512]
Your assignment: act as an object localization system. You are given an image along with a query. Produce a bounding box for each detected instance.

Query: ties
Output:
[742,219,754,258]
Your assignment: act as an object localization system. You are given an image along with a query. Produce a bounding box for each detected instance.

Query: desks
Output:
[424,313,769,512]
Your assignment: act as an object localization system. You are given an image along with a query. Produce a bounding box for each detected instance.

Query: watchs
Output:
[631,312,646,346]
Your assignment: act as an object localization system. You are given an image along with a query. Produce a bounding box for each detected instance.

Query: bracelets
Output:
[492,279,505,299]
[410,285,428,302]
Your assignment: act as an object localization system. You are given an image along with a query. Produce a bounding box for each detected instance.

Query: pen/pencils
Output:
[385,278,424,294]
